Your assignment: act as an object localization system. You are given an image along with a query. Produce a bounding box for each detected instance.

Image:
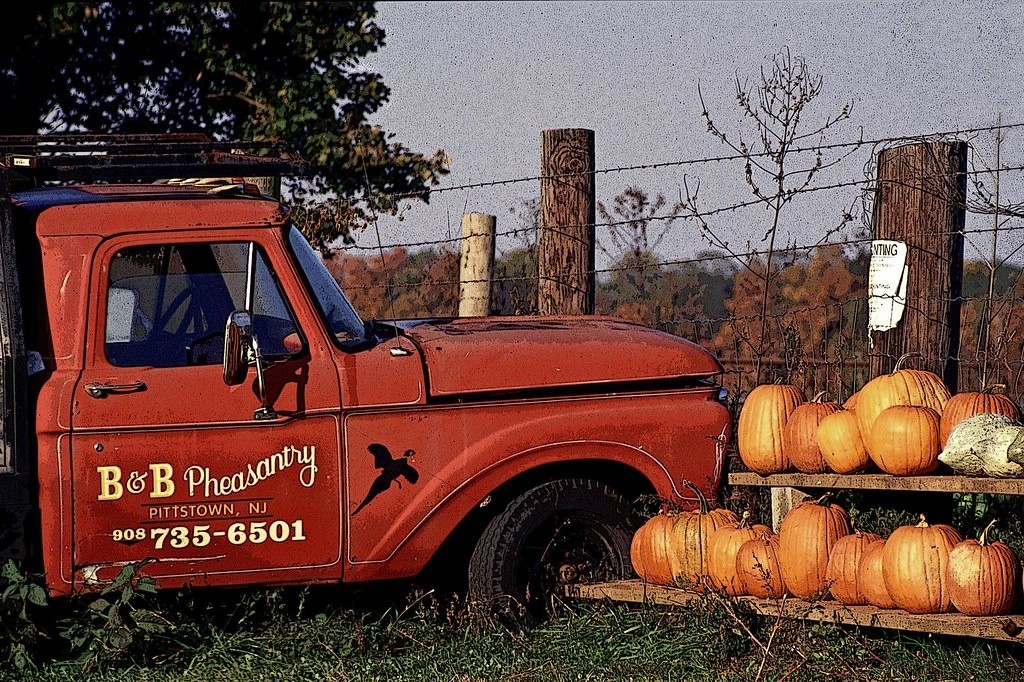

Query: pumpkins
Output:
[631,480,1021,616]
[737,351,1018,475]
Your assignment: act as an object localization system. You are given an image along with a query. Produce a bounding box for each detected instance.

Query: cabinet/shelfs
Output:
[564,472,1024,644]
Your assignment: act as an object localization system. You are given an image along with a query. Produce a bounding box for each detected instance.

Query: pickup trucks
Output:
[0,180,734,632]
